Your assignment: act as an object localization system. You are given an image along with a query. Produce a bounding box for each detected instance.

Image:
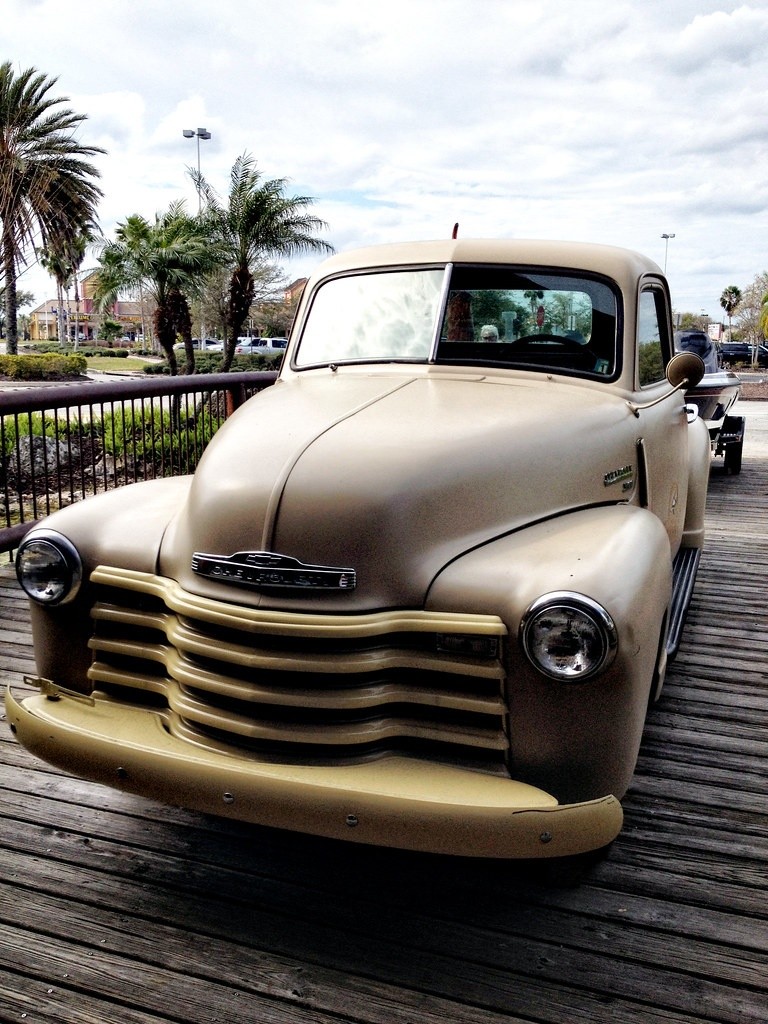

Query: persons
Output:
[480,324,499,342]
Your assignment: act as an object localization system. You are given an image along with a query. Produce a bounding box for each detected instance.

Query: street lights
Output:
[181,127,212,352]
[661,233,676,276]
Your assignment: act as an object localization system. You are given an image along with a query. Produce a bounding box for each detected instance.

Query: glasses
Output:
[481,335,498,341]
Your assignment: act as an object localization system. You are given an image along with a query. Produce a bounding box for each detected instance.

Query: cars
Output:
[120,337,130,342]
[670,330,719,373]
[76,334,87,341]
[5,236,720,860]
[205,335,288,355]
[173,338,222,350]
[134,334,147,342]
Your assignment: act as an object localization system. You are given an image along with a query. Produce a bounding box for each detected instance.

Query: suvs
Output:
[714,342,768,367]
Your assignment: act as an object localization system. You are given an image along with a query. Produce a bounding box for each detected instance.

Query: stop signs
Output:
[537,306,545,326]
